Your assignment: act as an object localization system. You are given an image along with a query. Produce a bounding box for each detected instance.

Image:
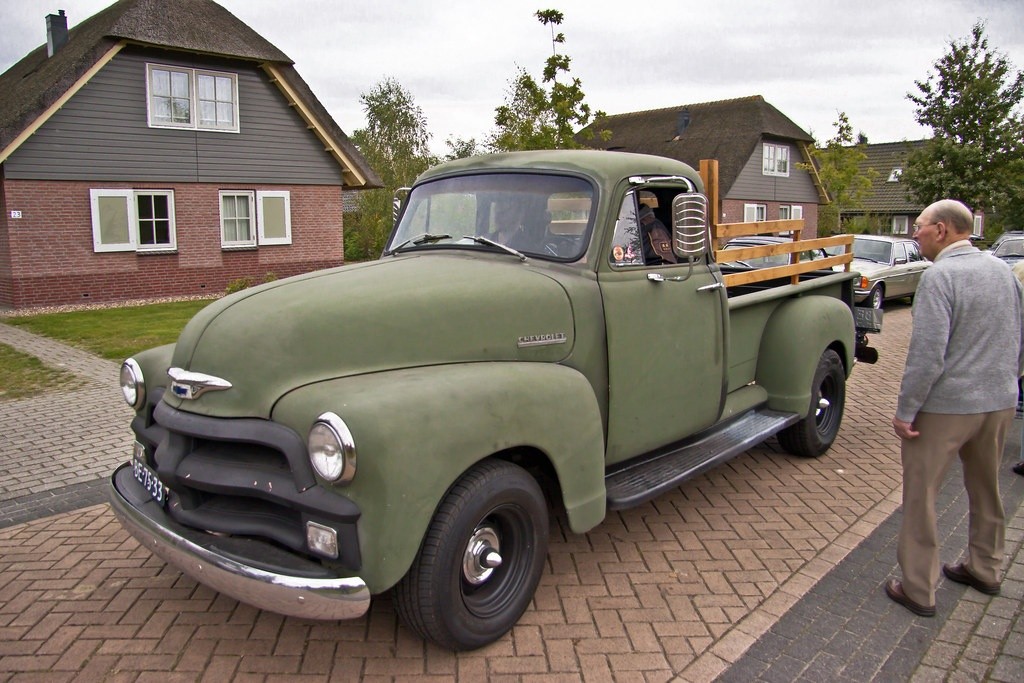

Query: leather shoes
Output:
[942,562,1002,595]
[885,577,935,616]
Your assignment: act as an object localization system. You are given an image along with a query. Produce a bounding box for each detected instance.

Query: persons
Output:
[886,199,1024,615]
[484,189,679,270]
[1011,259,1024,476]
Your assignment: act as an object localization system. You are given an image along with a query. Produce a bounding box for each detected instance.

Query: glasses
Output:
[912,221,935,231]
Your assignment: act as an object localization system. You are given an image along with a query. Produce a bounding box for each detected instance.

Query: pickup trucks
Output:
[108,149,883,654]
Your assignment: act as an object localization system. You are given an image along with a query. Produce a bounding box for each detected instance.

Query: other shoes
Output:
[1012,462,1024,475]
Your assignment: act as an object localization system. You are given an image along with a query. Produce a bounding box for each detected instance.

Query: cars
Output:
[995,235,1023,274]
[820,233,934,308]
[718,235,835,272]
